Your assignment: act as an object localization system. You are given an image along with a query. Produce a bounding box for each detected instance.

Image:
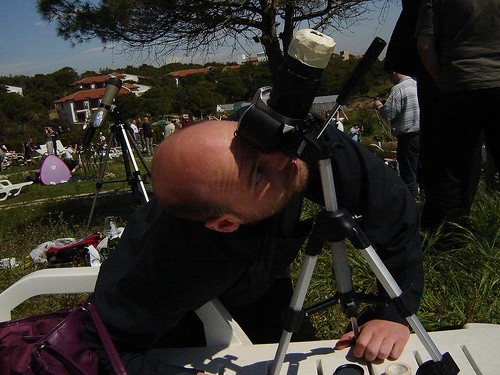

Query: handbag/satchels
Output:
[0,302,128,375]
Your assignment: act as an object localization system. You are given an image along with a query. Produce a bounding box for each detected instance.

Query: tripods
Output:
[268,36,463,375]
[84,121,151,231]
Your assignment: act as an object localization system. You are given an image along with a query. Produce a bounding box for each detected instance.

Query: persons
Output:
[413,0,500,252]
[81,103,423,375]
[374,63,421,199]
[0,110,364,172]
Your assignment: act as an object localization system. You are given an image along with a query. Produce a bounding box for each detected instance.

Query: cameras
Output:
[373,99,386,111]
[234,28,337,151]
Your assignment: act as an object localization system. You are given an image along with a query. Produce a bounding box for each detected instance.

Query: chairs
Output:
[0,267,258,347]
[0,179,34,202]
